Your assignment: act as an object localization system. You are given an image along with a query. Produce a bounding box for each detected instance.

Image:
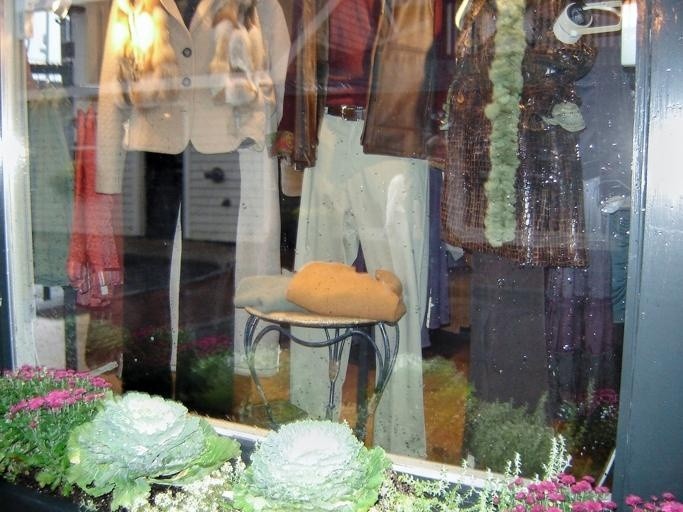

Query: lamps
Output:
[554,0,621,45]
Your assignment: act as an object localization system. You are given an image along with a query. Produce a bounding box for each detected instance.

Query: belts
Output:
[325,104,365,121]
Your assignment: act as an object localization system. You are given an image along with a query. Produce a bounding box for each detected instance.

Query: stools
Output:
[244,265,401,431]
